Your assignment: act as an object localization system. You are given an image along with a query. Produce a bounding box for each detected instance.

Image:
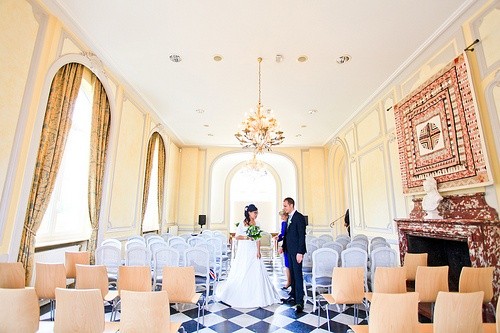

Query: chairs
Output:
[0,229,232,333]
[273,233,500,333]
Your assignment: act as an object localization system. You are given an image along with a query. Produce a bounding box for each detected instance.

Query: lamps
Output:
[234,57,286,155]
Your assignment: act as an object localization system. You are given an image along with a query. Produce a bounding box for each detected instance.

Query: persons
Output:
[422,176,443,219]
[344,209,350,237]
[216,204,280,309]
[274,197,308,315]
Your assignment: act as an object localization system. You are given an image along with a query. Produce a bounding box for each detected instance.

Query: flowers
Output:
[244,207,248,211]
[245,224,264,241]
[286,220,292,225]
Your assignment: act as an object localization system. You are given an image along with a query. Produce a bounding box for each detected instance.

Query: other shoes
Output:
[283,284,291,289]
[283,295,304,312]
